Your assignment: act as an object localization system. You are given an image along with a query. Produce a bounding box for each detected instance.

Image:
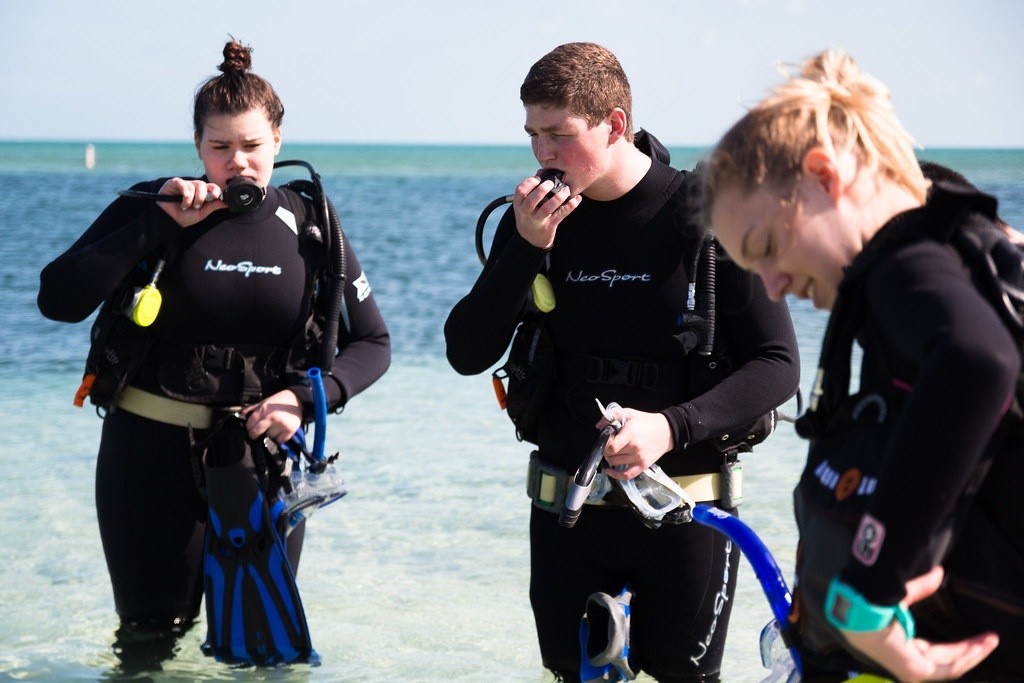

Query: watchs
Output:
[824,575,914,638]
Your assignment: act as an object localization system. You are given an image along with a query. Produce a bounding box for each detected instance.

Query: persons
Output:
[37,41,391,650]
[702,51,1023,682]
[444,44,801,676]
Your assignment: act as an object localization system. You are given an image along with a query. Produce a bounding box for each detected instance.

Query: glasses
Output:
[761,618,801,683]
[615,458,695,524]
[278,472,349,516]
